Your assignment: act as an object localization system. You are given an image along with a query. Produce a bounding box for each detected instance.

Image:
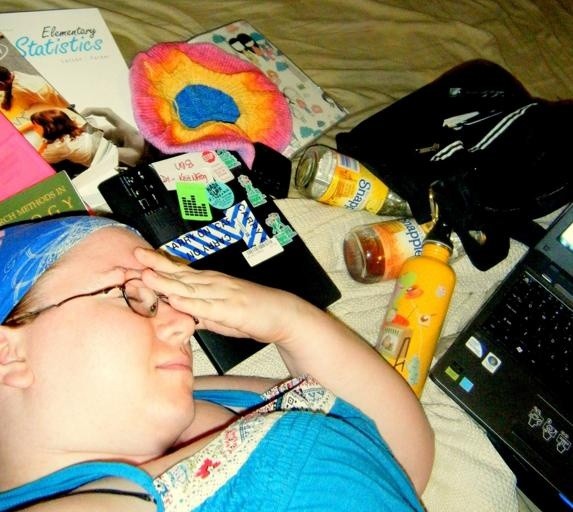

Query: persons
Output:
[0,216,435,511]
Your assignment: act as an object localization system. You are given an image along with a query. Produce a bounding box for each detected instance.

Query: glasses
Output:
[6,277,199,326]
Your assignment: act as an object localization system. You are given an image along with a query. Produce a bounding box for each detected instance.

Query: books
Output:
[186,18,350,161]
[0,6,170,229]
[96,148,343,377]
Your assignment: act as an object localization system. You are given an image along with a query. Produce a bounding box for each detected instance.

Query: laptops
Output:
[428,205,573,512]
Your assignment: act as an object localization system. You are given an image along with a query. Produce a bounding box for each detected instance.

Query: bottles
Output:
[344,211,467,285]
[371,215,455,400]
[293,143,414,217]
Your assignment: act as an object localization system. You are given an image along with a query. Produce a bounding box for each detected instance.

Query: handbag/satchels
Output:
[335,58,573,271]
